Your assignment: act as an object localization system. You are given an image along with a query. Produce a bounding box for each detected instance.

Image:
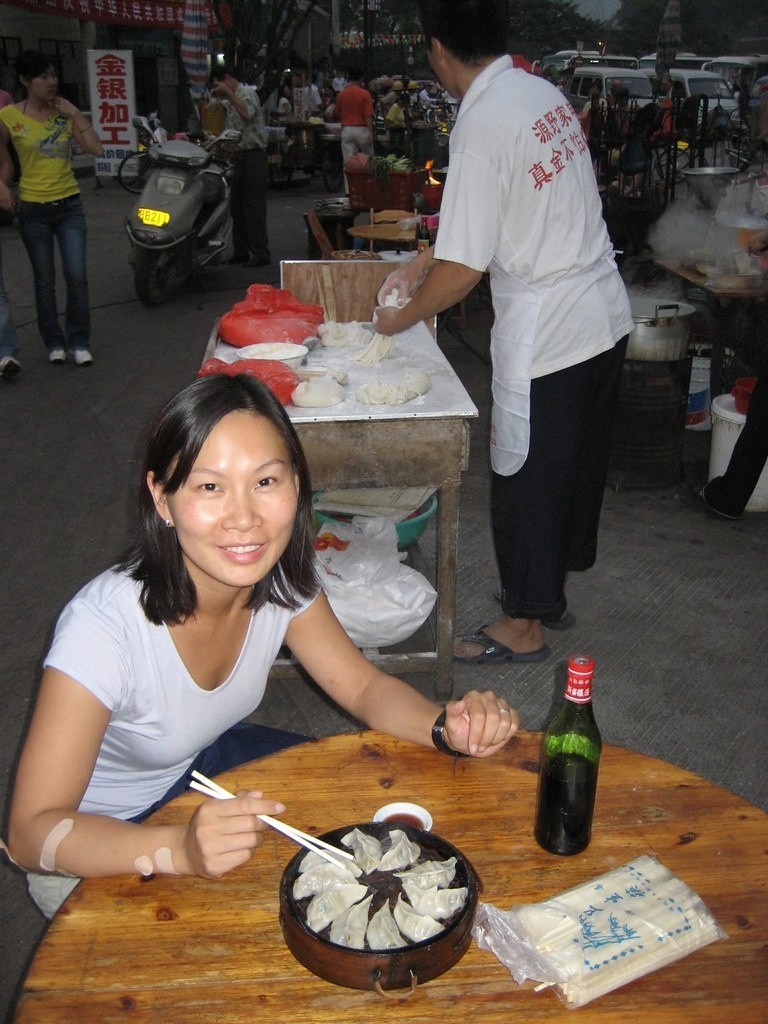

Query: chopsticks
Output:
[189,769,353,869]
[512,855,723,1008]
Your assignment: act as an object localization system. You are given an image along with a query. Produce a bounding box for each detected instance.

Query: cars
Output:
[543,49,768,185]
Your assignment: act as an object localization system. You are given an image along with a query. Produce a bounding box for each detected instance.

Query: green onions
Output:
[366,155,416,184]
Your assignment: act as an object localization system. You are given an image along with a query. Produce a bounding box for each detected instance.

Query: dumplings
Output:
[292,826,468,950]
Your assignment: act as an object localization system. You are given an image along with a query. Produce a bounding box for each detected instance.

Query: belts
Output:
[50,193,80,206]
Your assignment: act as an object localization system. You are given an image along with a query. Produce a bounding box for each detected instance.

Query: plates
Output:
[372,802,433,831]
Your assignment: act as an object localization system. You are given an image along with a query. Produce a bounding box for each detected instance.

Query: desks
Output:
[10,727,766,1024]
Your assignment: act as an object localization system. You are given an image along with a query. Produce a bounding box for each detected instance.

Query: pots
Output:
[623,299,696,362]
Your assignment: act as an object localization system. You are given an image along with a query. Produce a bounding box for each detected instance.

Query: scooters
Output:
[124,116,243,305]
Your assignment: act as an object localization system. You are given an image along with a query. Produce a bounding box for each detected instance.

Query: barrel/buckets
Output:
[327,123,342,133]
[707,393,768,513]
[684,356,712,432]
[604,353,692,487]
[421,184,444,213]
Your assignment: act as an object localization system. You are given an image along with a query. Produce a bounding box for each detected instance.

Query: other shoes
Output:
[222,256,250,264]
[243,258,271,267]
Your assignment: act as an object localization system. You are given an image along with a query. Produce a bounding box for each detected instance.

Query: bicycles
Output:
[118,111,205,195]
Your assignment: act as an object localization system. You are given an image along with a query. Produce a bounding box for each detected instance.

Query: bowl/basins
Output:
[237,342,308,370]
[673,247,717,275]
[681,166,740,192]
[310,490,438,549]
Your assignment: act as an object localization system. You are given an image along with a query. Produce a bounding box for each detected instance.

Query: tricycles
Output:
[263,81,460,194]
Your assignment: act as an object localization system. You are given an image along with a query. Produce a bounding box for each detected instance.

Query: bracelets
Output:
[431,710,469,757]
[79,124,90,133]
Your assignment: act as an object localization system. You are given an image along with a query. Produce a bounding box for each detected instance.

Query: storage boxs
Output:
[344,166,426,212]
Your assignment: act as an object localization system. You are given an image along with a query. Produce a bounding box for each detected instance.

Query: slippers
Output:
[494,587,576,631]
[454,629,552,664]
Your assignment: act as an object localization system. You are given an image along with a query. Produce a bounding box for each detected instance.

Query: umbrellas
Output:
[182,0,209,123]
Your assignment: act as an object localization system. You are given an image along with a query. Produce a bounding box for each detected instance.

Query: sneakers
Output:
[67,345,94,365]
[48,346,66,362]
[0,356,21,377]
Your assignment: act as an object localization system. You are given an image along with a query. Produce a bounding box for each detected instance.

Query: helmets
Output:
[408,82,420,89]
[393,81,403,90]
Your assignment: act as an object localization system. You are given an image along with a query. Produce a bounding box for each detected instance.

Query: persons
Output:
[0,49,104,378]
[277,70,460,197]
[8,374,520,919]
[575,80,673,196]
[210,67,271,267]
[374,0,635,660]
[703,229,768,518]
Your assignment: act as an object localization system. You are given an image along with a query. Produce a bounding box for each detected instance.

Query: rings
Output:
[500,708,510,713]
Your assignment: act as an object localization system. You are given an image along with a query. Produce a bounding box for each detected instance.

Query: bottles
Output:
[534,655,603,855]
[202,89,227,140]
[418,216,431,253]
